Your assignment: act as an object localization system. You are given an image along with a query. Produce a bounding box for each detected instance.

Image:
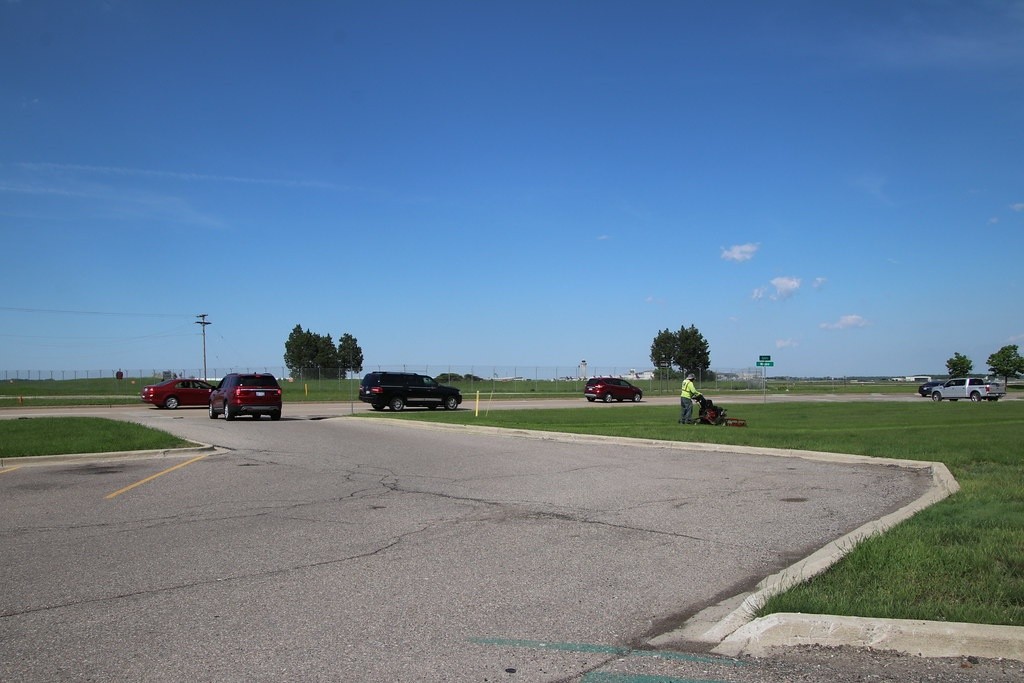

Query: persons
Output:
[679,374,703,424]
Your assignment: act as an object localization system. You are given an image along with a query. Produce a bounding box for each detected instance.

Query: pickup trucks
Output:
[929,377,1007,403]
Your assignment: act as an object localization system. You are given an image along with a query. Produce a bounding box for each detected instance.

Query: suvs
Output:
[208,373,282,422]
[358,370,464,412]
[584,377,642,403]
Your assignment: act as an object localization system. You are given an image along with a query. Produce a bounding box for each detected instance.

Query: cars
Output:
[918,381,949,397]
[142,378,218,410]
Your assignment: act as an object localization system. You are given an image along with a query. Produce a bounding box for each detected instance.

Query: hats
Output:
[686,373,696,380]
